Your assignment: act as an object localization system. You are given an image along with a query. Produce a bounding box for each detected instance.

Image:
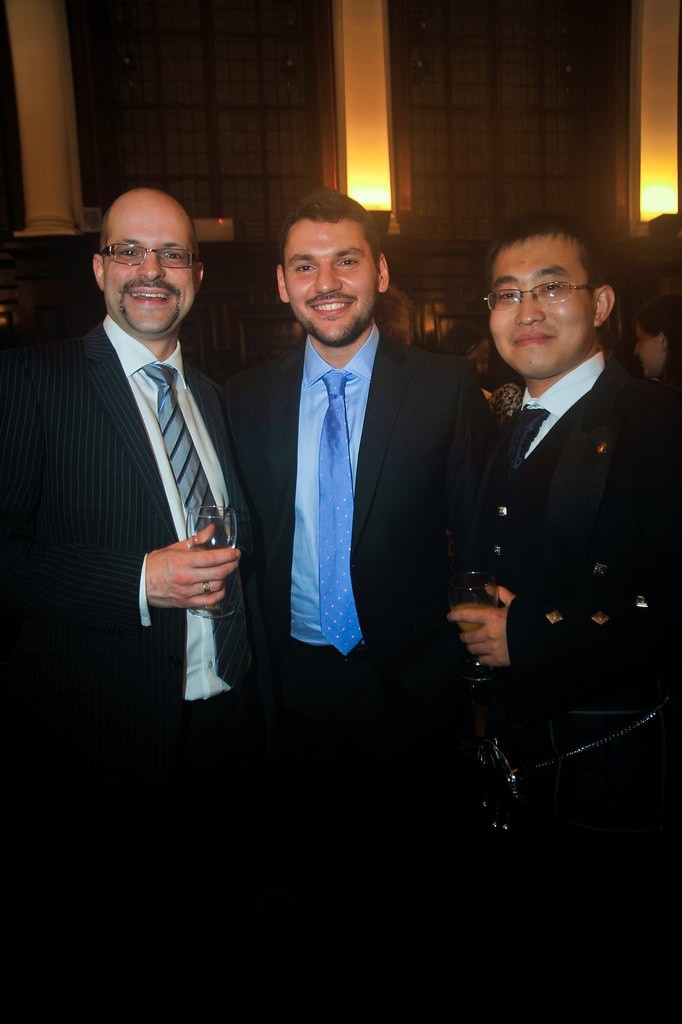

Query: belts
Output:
[280,636,374,665]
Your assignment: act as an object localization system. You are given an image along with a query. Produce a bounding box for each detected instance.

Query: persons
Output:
[1,188,254,1024]
[225,193,492,1023]
[374,208,682,1024]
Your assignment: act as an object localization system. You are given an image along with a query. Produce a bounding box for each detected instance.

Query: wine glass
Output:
[447,571,504,682]
[185,505,238,619]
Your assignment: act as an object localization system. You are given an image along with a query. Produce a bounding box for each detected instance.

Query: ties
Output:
[319,370,363,655]
[503,404,550,479]
[143,362,251,690]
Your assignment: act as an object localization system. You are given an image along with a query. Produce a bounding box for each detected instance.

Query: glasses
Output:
[483,281,594,310]
[100,242,195,269]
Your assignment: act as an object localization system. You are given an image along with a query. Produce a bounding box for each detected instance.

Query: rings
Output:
[204,583,210,595]
